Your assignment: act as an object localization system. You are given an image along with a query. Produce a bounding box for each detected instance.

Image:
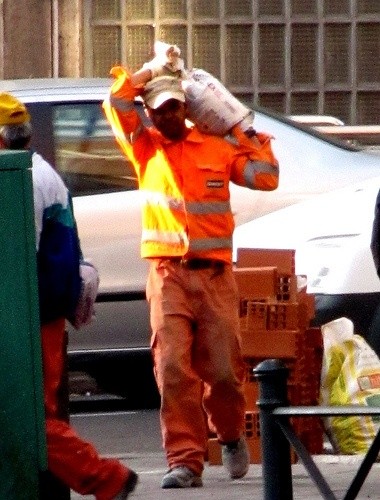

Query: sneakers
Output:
[221,435,250,477]
[162,465,203,488]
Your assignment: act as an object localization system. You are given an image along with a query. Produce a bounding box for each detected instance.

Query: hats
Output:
[142,74,187,110]
[0,92,30,125]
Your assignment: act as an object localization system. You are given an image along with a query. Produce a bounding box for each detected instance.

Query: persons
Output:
[101,40,279,491]
[0,93,141,500]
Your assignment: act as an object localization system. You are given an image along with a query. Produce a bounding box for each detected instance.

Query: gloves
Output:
[226,113,256,136]
[145,47,183,78]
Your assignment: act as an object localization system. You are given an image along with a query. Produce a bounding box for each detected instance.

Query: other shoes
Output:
[113,471,136,500]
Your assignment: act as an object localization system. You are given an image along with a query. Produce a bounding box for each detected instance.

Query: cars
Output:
[0,77,379,401]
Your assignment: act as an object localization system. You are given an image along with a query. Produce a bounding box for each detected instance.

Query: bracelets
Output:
[244,127,257,139]
